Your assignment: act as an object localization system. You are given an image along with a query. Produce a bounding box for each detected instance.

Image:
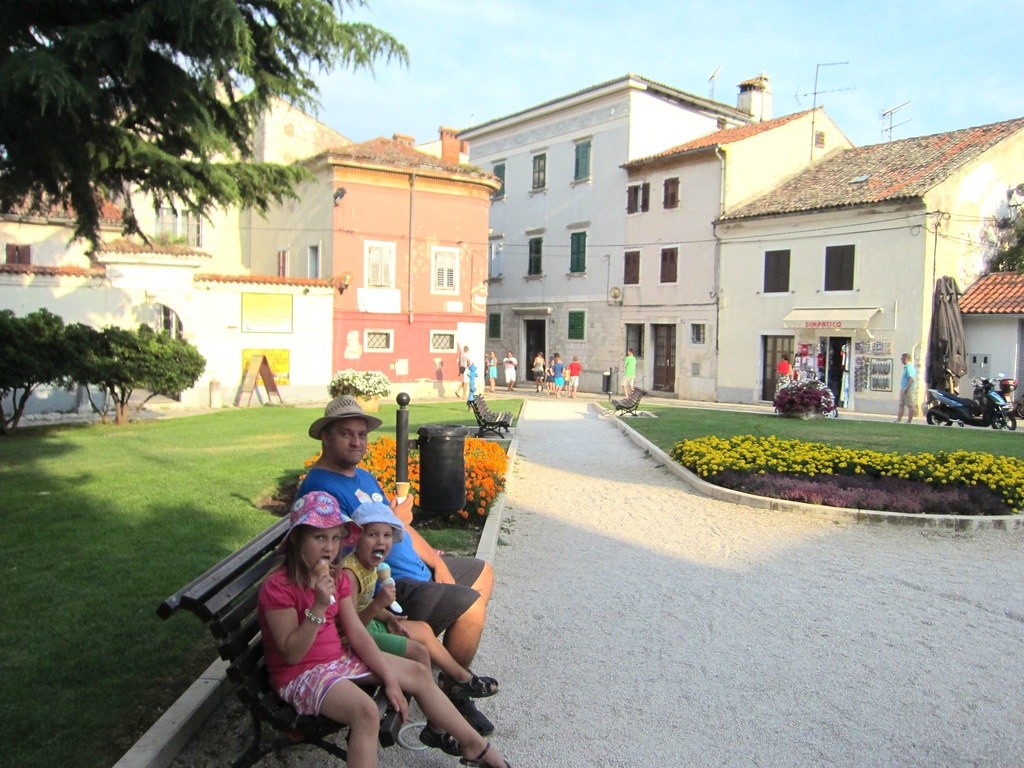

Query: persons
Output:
[257,489,511,768]
[567,355,582,399]
[293,395,496,737]
[773,353,794,414]
[893,352,917,423]
[454,345,471,400]
[621,349,637,400]
[331,500,500,758]
[545,352,571,399]
[485,351,518,393]
[531,351,546,393]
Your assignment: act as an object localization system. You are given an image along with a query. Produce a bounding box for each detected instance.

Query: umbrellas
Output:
[931,276,968,393]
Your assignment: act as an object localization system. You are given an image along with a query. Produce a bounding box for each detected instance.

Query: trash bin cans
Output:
[418,424,469,513]
[603,372,611,392]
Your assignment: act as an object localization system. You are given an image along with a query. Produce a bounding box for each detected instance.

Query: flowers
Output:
[773,383,837,415]
[327,368,392,402]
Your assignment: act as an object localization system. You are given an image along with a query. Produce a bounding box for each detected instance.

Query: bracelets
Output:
[304,608,327,624]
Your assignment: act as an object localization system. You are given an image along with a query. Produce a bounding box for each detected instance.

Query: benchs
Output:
[467,393,515,439]
[611,387,646,416]
[157,511,412,768]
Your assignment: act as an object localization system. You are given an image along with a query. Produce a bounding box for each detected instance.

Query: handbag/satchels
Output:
[460,367,465,374]
[534,363,544,372]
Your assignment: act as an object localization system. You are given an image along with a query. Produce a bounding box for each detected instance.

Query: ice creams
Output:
[377,562,391,583]
[395,482,410,497]
[315,559,329,577]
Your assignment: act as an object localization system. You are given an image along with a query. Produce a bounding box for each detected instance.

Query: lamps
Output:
[610,287,623,307]
[336,272,353,295]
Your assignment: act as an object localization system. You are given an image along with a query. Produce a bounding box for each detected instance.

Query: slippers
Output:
[460,742,510,768]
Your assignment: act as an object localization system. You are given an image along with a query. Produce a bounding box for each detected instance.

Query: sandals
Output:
[418,726,464,756]
[443,672,500,698]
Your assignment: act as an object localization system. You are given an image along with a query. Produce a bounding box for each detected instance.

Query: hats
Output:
[351,501,404,545]
[309,397,383,440]
[275,490,364,553]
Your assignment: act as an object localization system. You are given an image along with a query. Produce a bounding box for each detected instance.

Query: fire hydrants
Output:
[467,363,478,411]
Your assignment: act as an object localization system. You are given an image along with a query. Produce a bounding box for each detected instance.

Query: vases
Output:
[777,404,816,421]
[347,394,380,413]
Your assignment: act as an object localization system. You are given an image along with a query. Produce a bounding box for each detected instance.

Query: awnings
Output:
[783,309,879,329]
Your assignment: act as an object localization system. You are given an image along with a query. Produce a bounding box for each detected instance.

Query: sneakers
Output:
[451,696,495,736]
[437,667,476,690]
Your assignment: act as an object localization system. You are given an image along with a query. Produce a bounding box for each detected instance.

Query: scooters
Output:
[926,373,1018,431]
[1016,394,1024,419]
[775,406,838,420]
